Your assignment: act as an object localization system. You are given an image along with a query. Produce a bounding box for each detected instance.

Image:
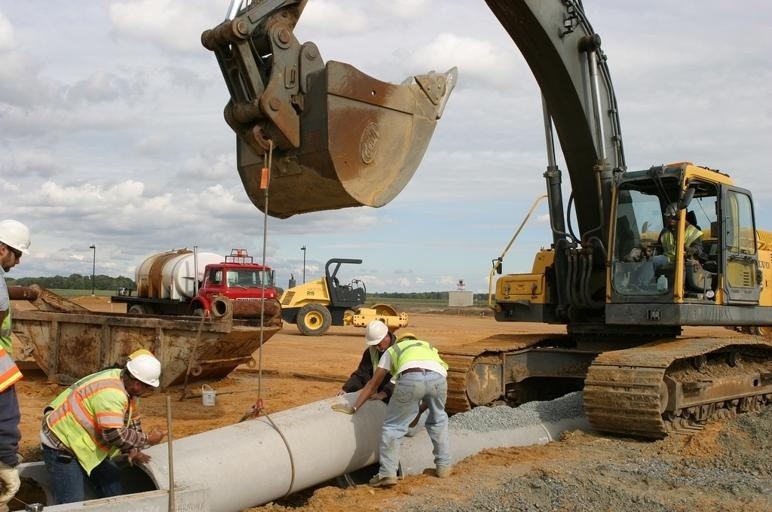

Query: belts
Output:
[399,368,432,376]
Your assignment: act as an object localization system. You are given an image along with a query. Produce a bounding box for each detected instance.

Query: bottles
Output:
[657,274,667,291]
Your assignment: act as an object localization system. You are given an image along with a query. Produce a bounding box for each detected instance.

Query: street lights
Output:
[90,245,96,294]
[301,244,306,284]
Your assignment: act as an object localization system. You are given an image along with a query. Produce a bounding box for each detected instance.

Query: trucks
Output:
[110,249,280,319]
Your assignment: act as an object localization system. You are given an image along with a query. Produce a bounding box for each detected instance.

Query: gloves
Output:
[332,405,354,414]
[0,462,20,504]
[409,412,422,427]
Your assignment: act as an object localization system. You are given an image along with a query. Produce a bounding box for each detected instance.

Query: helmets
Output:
[395,333,418,343]
[664,203,687,217]
[0,220,32,256]
[365,321,388,346]
[126,349,161,388]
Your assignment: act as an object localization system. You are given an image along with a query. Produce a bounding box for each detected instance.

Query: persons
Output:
[0,345,25,512]
[40,355,162,504]
[336,320,399,404]
[0,220,32,357]
[628,202,704,292]
[331,332,451,487]
[42,348,155,415]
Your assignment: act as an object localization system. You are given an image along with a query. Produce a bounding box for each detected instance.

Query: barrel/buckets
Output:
[202,384,216,407]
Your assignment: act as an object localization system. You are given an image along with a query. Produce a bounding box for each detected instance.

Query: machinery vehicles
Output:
[279,256,409,336]
[200,0,772,439]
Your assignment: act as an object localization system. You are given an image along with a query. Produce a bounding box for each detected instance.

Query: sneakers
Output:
[368,473,398,487]
[436,464,462,477]
[628,283,648,293]
